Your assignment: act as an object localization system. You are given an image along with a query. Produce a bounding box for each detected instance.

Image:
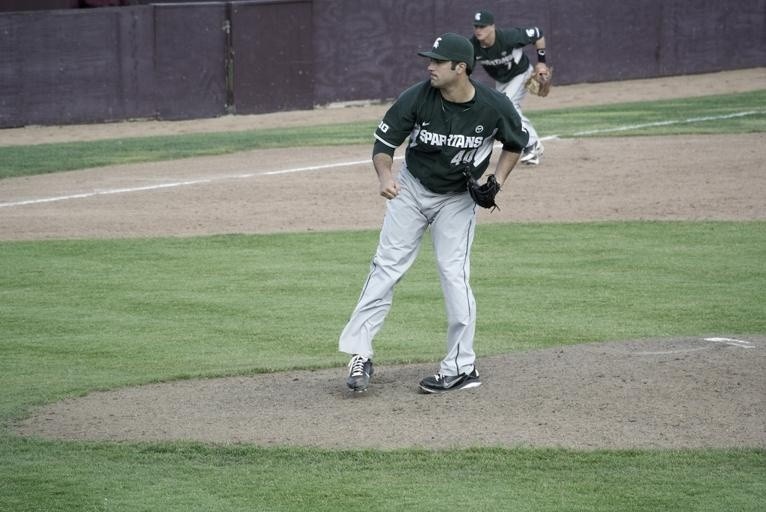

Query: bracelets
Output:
[536,48,547,66]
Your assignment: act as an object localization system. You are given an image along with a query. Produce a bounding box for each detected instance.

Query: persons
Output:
[336,32,530,396]
[469,10,551,164]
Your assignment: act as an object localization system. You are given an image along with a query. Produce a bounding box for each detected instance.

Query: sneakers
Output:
[419,365,482,394]
[346,354,373,393]
[520,140,544,166]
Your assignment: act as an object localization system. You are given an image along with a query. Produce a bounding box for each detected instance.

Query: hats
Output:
[472,10,494,26]
[417,33,474,70]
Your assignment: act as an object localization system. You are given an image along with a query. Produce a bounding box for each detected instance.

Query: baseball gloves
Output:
[462,165,501,207]
[525,66,554,96]
